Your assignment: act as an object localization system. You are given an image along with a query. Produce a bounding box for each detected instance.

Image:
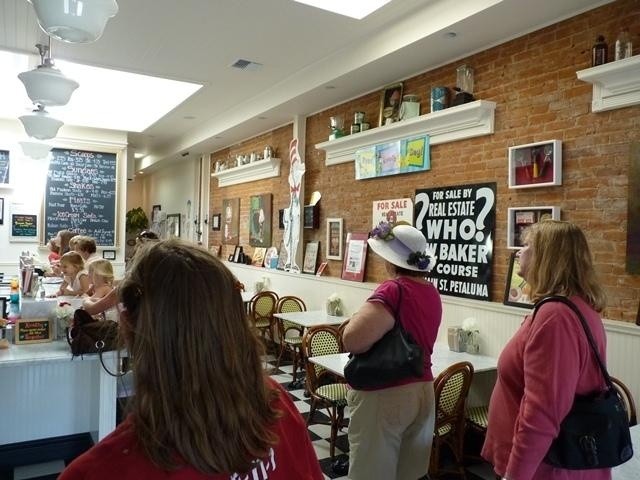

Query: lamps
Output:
[16,36,81,108]
[16,141,54,162]
[16,105,65,140]
[24,0,121,46]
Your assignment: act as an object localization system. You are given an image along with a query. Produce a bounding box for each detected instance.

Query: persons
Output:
[57,236,327,480]
[136,231,159,247]
[476,219,635,480]
[43,231,120,323]
[338,220,446,480]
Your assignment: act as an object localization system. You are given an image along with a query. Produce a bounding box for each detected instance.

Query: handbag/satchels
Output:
[69,309,126,357]
[344,327,425,390]
[543,387,633,470]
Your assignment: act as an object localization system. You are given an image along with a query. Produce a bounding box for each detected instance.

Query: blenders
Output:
[327,115,345,141]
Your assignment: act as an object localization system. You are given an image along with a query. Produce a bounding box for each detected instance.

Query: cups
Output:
[10,294,18,304]
[384,118,394,125]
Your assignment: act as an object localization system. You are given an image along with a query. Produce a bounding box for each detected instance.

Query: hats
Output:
[367,220,437,271]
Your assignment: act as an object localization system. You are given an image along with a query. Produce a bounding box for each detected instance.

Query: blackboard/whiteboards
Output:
[8,212,38,243]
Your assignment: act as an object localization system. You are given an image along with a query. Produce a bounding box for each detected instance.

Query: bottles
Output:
[255,278,270,295]
[333,297,343,315]
[401,94,421,121]
[592,33,608,69]
[614,27,633,61]
[32,272,45,301]
[211,145,274,172]
[463,329,481,354]
[5,322,14,345]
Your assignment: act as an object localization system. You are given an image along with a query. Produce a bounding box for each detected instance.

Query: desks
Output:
[0,272,129,449]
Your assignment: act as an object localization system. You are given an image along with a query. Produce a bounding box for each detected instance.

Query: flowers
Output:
[404,250,433,270]
[366,220,412,243]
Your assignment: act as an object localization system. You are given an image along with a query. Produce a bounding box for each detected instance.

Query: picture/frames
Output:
[211,213,221,231]
[167,213,182,237]
[301,203,370,282]
[227,244,251,265]
[152,204,162,221]
[277,208,286,230]
[0,197,5,225]
[209,243,222,259]
[0,145,15,190]
[276,239,288,270]
[503,252,539,309]
[103,249,116,260]
[0,296,8,340]
[8,204,40,243]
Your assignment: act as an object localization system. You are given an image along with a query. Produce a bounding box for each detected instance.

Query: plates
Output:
[264,247,277,268]
